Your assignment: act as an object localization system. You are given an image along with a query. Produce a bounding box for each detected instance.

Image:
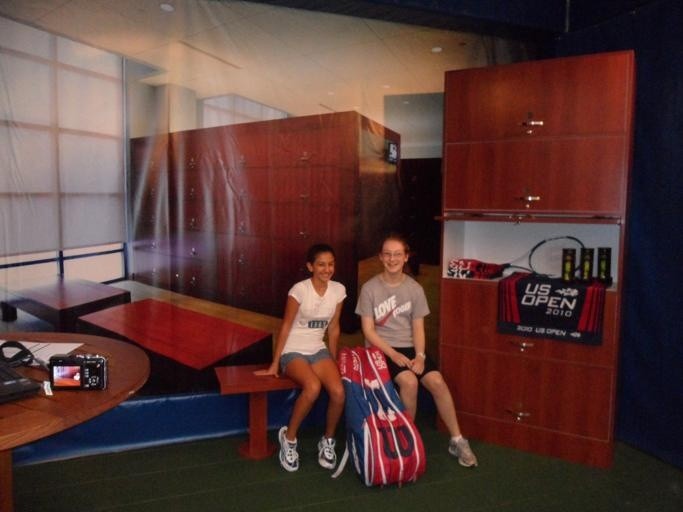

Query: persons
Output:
[353,231,479,468]
[251,241,346,473]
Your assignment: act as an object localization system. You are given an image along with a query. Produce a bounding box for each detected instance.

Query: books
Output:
[0,338,86,367]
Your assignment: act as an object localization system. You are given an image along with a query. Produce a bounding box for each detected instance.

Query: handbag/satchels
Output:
[336,343,427,488]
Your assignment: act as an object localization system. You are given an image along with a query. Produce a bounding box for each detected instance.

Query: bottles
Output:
[562,247,612,284]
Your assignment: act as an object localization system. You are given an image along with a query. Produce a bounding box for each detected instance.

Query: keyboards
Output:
[0,362,41,403]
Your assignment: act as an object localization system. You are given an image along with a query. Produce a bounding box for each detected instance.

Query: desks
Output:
[0,332,151,511]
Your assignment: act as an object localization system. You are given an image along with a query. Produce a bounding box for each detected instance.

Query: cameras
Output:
[49,354,108,391]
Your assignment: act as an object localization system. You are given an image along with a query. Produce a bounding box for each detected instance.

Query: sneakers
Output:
[276,424,301,473]
[446,436,478,468]
[314,433,336,471]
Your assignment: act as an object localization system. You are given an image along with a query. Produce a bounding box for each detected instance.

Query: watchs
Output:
[413,351,427,360]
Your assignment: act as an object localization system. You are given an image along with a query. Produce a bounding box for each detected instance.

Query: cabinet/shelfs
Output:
[129,111,401,335]
[434,47,636,468]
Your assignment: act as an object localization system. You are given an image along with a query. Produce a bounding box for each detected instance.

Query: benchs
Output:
[215,363,303,458]
[0,273,273,392]
